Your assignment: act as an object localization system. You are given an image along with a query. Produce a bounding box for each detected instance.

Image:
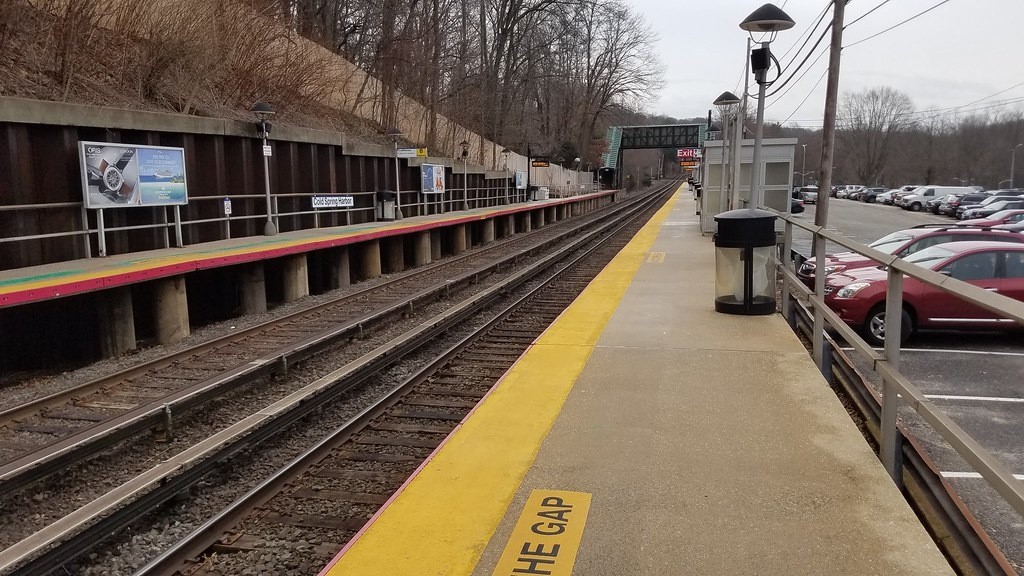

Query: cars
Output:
[795,223,1024,293]
[806,185,817,188]
[830,184,1024,233]
[818,239,1024,348]
[792,186,800,198]
[797,186,819,204]
[791,198,805,213]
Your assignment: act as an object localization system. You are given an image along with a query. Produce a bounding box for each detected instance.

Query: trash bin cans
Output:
[688,177,702,215]
[713,208,780,317]
[377,190,397,222]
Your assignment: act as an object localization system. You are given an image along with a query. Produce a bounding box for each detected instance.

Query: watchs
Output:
[98,149,134,192]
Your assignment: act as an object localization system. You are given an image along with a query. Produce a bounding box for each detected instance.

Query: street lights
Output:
[459,140,471,210]
[739,3,796,212]
[598,167,606,192]
[792,145,814,187]
[1010,143,1023,189]
[998,180,1012,189]
[527,141,541,200]
[501,148,512,205]
[713,91,742,215]
[952,178,975,186]
[388,128,404,220]
[557,156,566,198]
[575,157,581,196]
[249,102,278,237]
